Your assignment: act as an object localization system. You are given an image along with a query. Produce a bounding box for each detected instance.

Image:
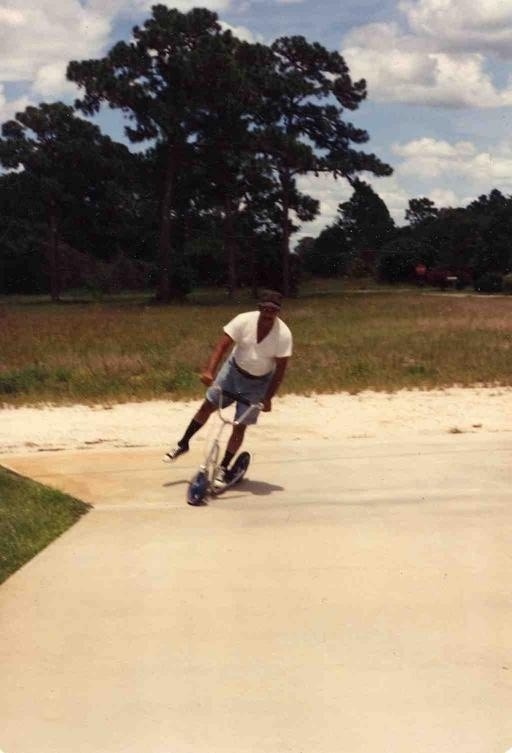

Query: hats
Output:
[256,289,282,309]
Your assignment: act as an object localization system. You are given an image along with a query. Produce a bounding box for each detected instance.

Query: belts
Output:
[231,356,266,379]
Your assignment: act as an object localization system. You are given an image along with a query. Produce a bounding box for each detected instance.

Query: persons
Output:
[162,288,295,491]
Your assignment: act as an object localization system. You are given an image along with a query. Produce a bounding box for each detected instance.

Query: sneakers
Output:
[214,466,227,490]
[162,445,188,463]
